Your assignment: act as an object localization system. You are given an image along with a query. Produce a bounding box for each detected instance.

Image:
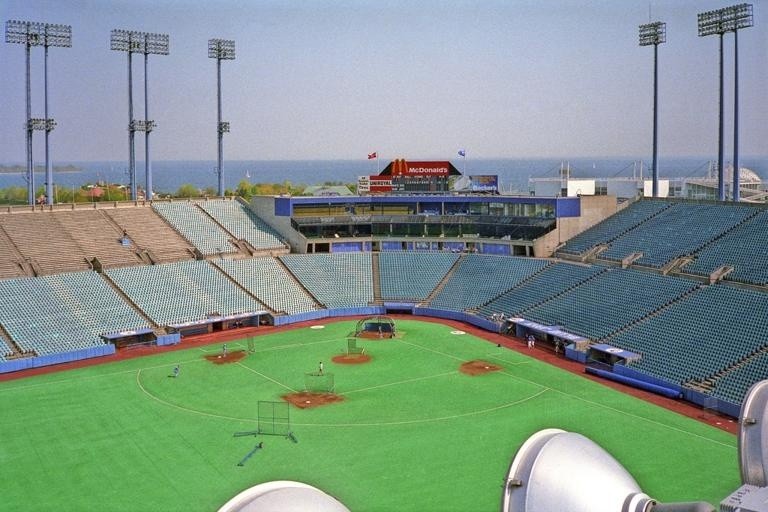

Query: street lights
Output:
[111,28,170,203]
[695,5,753,202]
[206,39,236,200]
[5,20,73,208]
[638,21,667,201]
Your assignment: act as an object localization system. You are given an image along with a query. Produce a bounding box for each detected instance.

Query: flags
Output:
[457,149,465,157]
[367,151,376,160]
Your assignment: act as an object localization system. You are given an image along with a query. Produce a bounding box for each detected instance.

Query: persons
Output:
[377,326,383,339]
[172,366,179,378]
[524,332,536,350]
[484,310,523,338]
[317,361,324,376]
[222,343,227,357]
[551,339,570,356]
[123,229,128,240]
